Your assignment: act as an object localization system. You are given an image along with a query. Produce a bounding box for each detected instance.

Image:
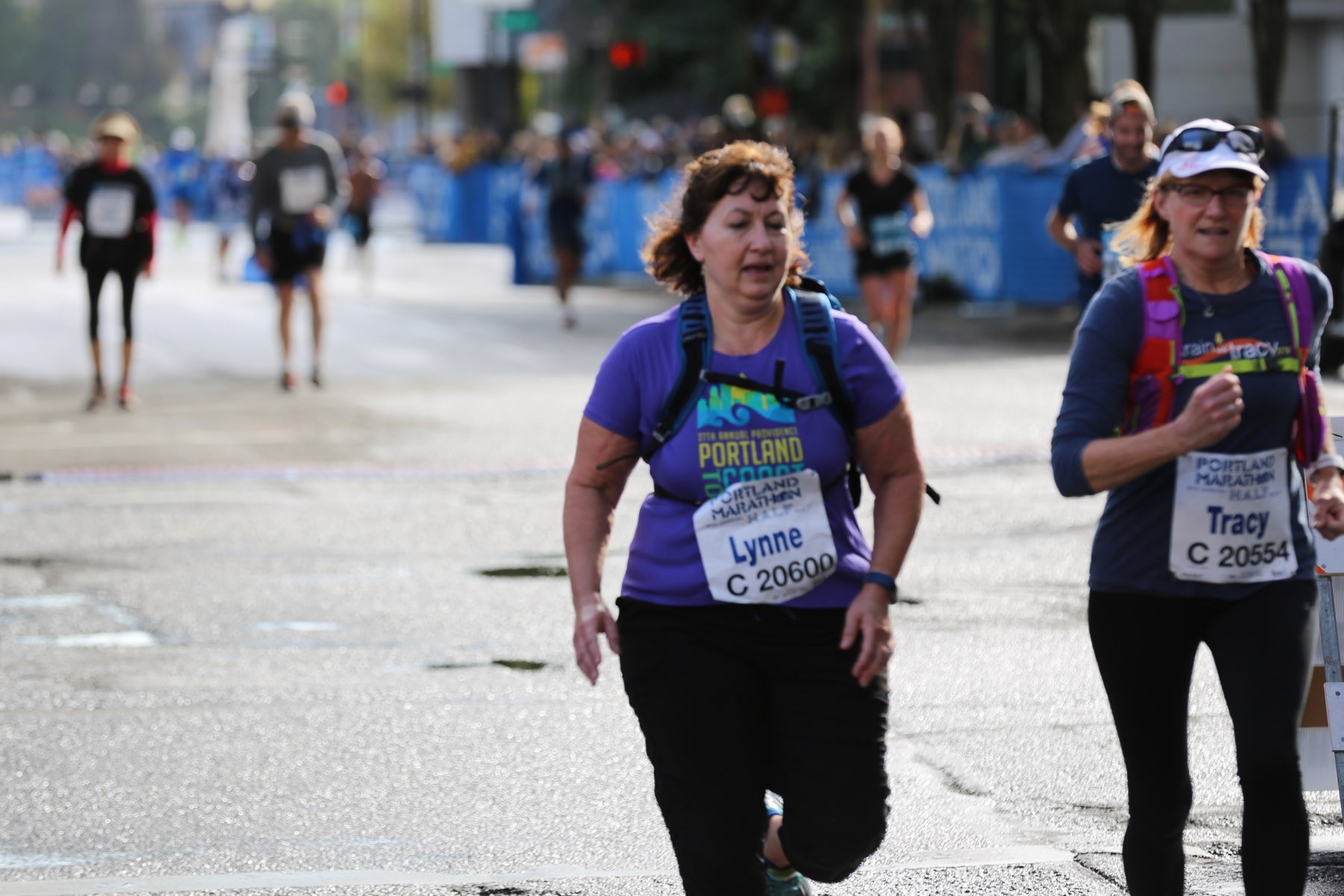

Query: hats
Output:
[90,112,135,139]
[1157,117,1271,181]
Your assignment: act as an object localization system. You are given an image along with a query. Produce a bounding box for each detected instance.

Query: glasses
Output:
[1164,183,1251,204]
[1161,125,1262,161]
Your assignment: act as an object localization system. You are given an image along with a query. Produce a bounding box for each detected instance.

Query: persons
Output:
[163,122,248,289]
[834,120,935,362]
[1048,84,1164,325]
[1049,115,1344,896]
[526,133,603,331]
[251,90,352,389]
[342,136,382,295]
[52,111,159,416]
[561,141,926,896]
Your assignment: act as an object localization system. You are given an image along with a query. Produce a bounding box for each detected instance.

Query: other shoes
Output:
[284,372,297,388]
[754,791,808,896]
[311,367,322,383]
[87,389,105,407]
[119,388,131,405]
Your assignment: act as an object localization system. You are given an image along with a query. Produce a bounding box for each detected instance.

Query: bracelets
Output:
[1305,453,1343,485]
[859,571,898,606]
